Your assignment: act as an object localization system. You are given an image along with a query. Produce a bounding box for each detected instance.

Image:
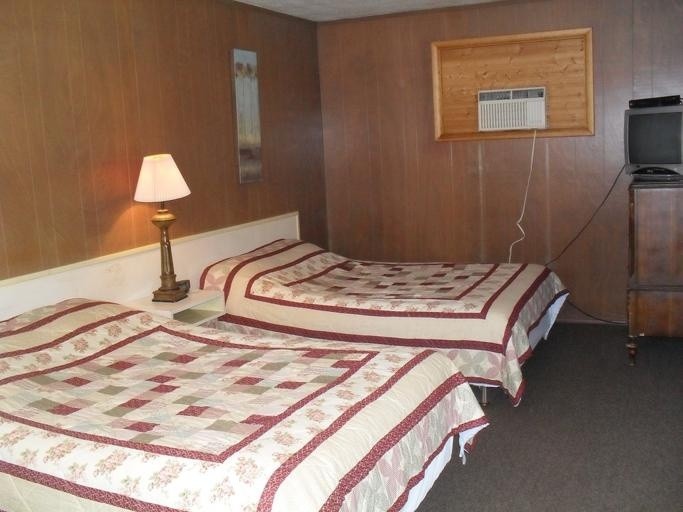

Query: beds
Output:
[0,296,491,512]
[198,238,572,407]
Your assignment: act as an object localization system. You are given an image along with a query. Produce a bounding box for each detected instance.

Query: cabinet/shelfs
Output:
[625,181,683,366]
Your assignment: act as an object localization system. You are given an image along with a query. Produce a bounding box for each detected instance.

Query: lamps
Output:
[133,153,191,302]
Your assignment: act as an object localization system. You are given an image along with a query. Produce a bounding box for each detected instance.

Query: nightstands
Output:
[124,288,228,329]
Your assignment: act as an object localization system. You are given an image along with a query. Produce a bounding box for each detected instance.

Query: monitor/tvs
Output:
[623,106,683,182]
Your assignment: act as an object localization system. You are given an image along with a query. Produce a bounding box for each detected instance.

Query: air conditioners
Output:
[477,86,546,133]
